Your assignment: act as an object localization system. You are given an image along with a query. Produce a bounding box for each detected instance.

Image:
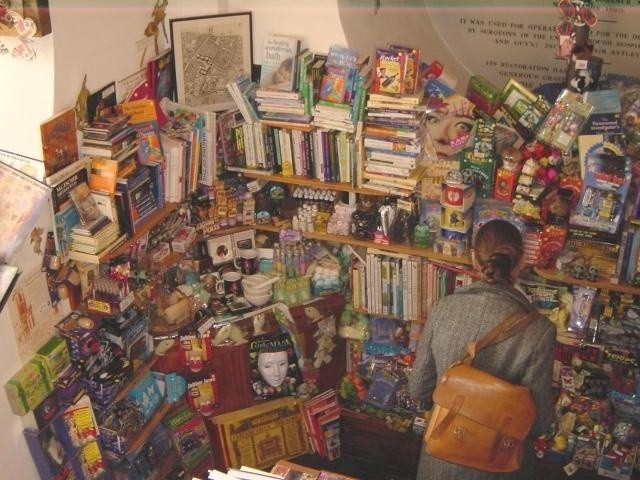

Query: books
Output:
[351,251,444,322]
[68,36,460,260]
[207,388,341,478]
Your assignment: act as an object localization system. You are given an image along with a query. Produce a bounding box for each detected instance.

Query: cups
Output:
[233,250,258,275]
[216,272,242,296]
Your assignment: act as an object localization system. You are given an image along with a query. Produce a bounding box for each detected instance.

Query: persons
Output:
[406,220,556,480]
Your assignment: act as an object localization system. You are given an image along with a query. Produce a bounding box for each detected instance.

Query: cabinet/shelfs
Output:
[42,109,640,479]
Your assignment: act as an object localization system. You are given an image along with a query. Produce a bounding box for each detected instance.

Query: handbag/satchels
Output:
[419,306,544,475]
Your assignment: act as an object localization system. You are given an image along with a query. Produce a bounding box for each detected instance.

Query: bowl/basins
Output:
[243,274,272,306]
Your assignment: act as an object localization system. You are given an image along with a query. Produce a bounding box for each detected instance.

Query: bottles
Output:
[495,148,522,202]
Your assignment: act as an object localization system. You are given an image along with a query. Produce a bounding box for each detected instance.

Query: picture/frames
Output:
[166,9,256,111]
